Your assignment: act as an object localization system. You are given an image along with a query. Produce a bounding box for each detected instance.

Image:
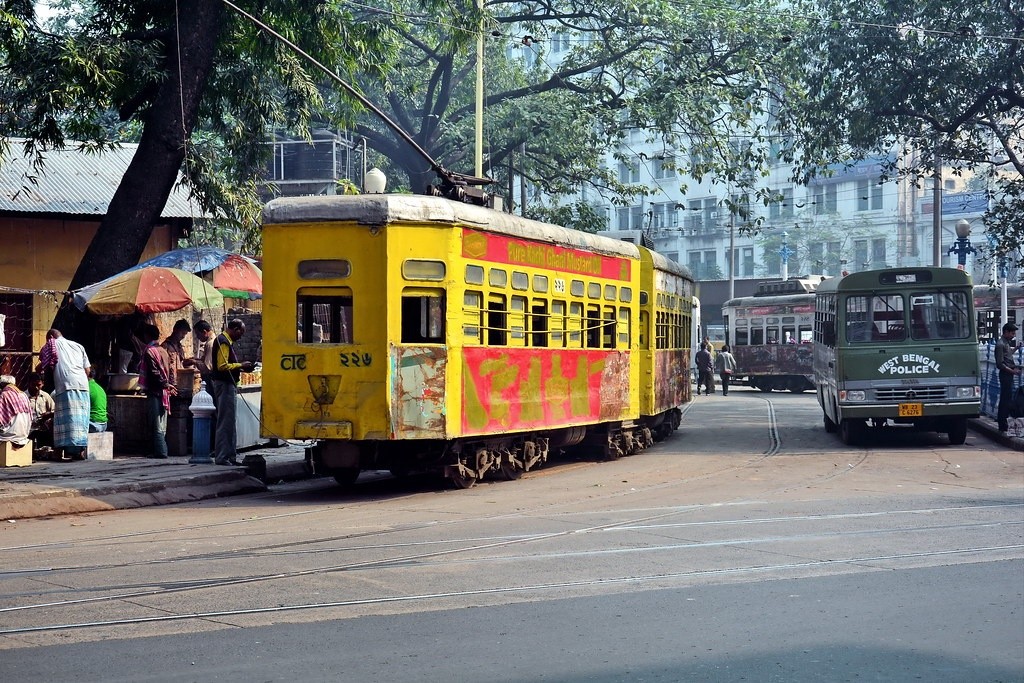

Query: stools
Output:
[85,432,113,461]
[0,440,32,467]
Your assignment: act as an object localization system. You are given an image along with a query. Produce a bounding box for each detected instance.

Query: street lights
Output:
[947,218,979,270]
[781,231,791,281]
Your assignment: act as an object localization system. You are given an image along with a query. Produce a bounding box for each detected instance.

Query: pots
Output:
[107,372,143,392]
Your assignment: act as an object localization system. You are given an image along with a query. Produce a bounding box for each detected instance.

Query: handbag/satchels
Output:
[723,368,732,373]
[42,365,55,395]
[119,348,133,374]
[1008,385,1024,417]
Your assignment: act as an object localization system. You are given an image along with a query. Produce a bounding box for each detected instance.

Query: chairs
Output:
[847,321,957,345]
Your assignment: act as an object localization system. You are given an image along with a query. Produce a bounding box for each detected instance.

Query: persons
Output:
[716,345,736,396]
[994,323,1022,432]
[695,336,716,395]
[138,318,252,466]
[0,328,108,463]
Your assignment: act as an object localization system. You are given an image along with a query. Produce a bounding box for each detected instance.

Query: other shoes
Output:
[706,394,710,395]
[999,426,1006,430]
[209,451,215,456]
[233,462,242,465]
[147,454,167,458]
[216,460,232,465]
[697,387,701,395]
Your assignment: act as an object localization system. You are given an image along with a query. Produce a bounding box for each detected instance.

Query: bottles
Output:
[235,369,262,385]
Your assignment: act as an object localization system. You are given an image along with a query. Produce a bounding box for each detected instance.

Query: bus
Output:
[971,281,1024,424]
[812,265,983,445]
[720,274,946,396]
[210,0,700,491]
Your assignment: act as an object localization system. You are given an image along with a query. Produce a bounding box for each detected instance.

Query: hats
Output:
[0,375,15,384]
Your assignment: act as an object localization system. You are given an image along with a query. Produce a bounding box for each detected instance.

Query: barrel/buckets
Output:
[176,368,195,390]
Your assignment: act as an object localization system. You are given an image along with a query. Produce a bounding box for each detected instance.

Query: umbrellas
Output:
[73,246,262,323]
